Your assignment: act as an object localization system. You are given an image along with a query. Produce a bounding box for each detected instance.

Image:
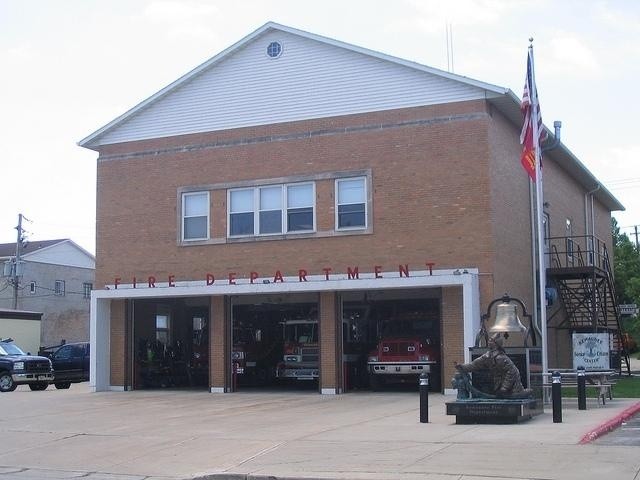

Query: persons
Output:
[456,334,524,397]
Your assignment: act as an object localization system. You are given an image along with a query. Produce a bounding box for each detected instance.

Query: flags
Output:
[519,50,550,183]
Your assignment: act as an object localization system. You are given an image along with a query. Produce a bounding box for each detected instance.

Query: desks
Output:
[543,369,619,404]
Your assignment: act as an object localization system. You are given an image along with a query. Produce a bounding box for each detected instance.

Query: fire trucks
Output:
[276,307,364,390]
[366,307,441,390]
[185,308,272,388]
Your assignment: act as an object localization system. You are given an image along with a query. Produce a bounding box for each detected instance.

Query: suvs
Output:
[0,337,53,392]
[30,340,91,390]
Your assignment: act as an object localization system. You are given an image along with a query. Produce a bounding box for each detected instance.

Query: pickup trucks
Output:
[612,331,637,355]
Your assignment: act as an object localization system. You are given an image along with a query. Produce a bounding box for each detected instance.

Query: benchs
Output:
[551,382,615,400]
[544,385,611,407]
